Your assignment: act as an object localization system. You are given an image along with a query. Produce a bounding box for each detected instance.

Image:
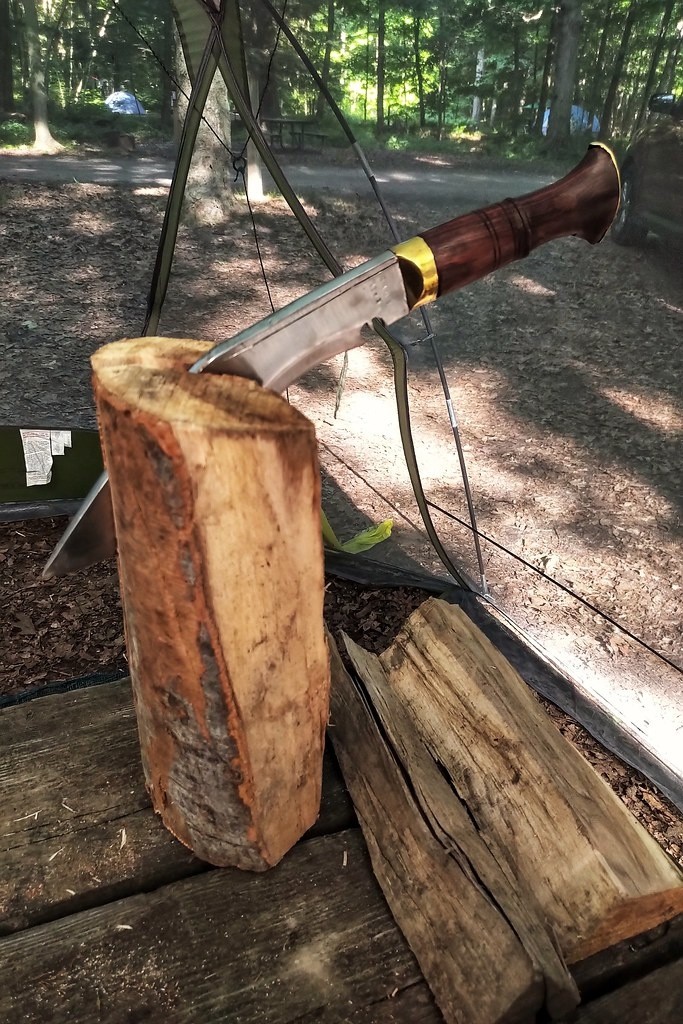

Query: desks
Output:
[261,118,317,153]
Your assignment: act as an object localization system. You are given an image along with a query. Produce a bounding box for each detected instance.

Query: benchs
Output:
[289,130,330,154]
[262,131,282,154]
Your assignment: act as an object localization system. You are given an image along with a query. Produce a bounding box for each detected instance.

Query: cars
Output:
[610,91,683,247]
